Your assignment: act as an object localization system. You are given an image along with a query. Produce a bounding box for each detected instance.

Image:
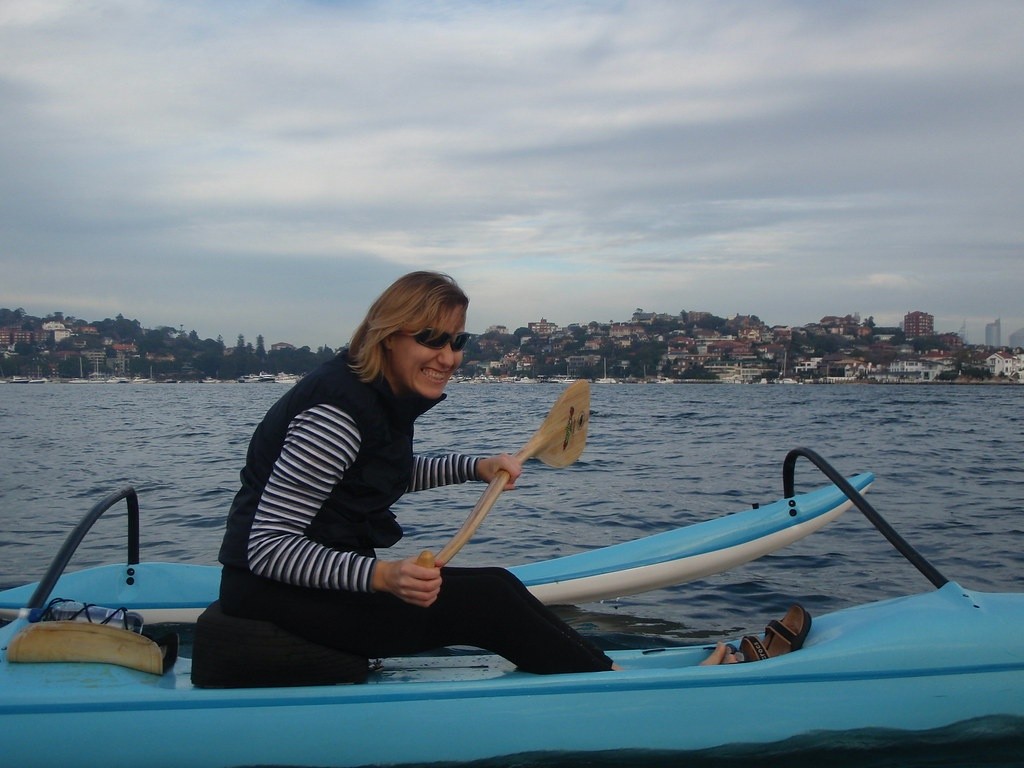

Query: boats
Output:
[0,444,1022,768]
[0,355,300,385]
[448,355,675,385]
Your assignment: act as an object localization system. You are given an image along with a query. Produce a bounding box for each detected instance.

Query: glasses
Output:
[416,328,470,352]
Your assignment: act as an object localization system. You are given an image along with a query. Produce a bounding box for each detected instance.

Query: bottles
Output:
[26,601,144,636]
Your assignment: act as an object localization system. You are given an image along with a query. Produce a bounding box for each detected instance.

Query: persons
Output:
[218,270,742,674]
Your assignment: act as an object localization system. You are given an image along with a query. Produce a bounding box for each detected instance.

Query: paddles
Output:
[417,378,592,568]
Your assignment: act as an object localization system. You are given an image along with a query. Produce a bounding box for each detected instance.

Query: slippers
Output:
[727,603,812,663]
